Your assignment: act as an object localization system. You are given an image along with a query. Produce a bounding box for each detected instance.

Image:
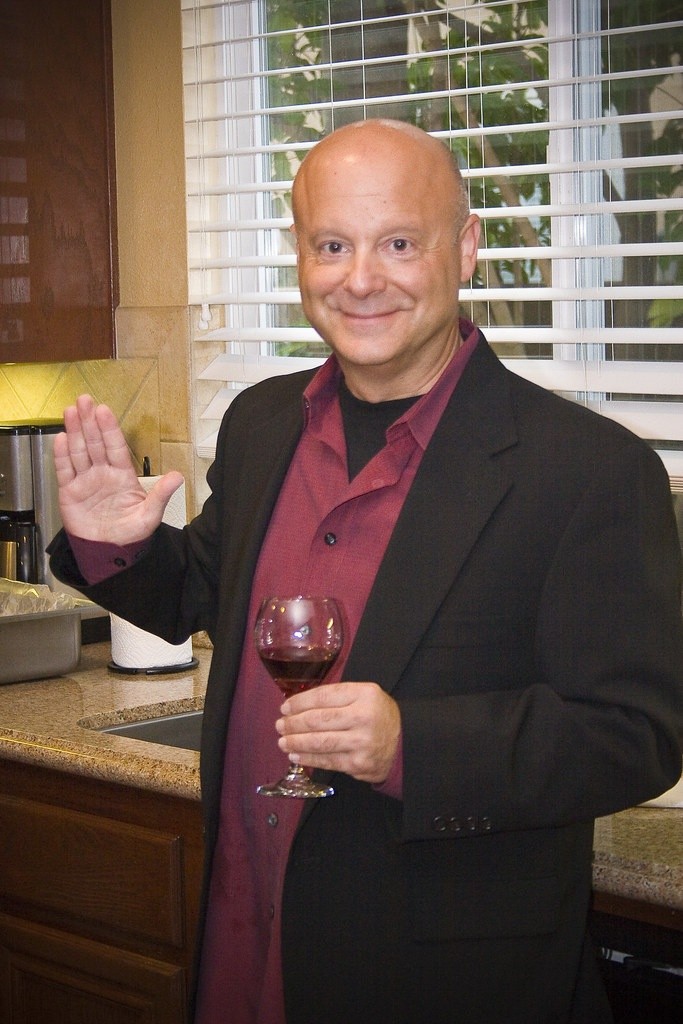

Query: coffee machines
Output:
[0,417,109,619]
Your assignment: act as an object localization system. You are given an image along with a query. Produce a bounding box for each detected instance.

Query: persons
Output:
[47,118,682,1024]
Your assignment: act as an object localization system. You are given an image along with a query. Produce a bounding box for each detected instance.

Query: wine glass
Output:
[253,597,344,799]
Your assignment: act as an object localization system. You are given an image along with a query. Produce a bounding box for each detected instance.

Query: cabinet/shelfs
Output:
[0,762,210,1024]
[0,1,121,363]
